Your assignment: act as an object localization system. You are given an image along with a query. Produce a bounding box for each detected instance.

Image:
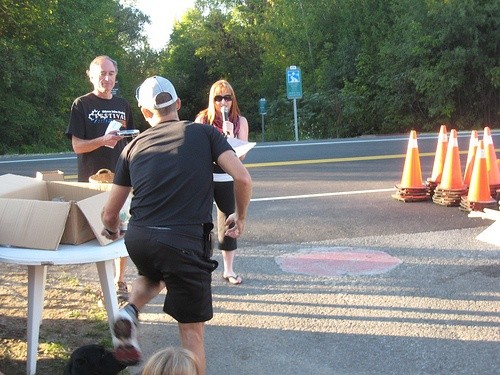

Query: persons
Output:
[142,347,201,375]
[194,79,248,284]
[65,55,136,308]
[102,75,253,375]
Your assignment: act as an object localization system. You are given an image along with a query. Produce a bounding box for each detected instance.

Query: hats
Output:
[136,76,178,110]
[64,344,128,375]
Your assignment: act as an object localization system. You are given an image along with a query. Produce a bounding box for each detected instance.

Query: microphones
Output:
[220,106,229,134]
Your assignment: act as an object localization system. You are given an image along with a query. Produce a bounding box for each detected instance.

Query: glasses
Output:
[214,94,232,102]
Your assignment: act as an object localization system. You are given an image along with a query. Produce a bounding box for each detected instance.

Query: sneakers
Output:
[113,302,141,366]
[116,281,128,304]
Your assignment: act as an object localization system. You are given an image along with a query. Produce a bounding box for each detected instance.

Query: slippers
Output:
[223,275,242,284]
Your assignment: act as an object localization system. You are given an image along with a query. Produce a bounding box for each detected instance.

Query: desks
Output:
[0,240,128,375]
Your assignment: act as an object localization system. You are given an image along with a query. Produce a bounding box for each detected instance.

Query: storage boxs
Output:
[35,170,64,181]
[0,172,125,250]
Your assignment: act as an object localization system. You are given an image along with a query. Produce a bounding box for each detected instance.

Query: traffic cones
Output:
[432,128,468,207]
[464,129,481,190]
[393,130,432,202]
[427,124,449,198]
[458,140,499,214]
[483,126,500,202]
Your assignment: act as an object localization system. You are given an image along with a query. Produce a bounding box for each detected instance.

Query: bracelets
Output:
[105,228,118,236]
